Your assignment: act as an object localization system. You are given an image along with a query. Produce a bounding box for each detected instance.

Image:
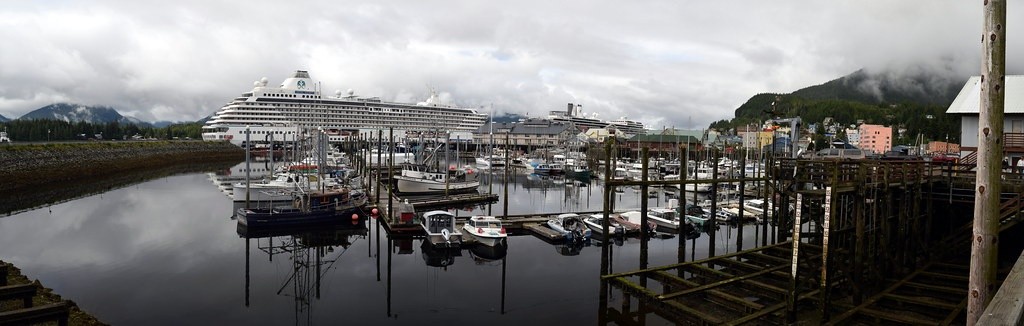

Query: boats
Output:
[207,144,481,229]
[421,210,463,248]
[676,205,711,227]
[702,207,737,221]
[647,209,690,229]
[475,148,809,220]
[463,215,507,247]
[611,211,657,234]
[583,213,626,235]
[547,213,592,243]
